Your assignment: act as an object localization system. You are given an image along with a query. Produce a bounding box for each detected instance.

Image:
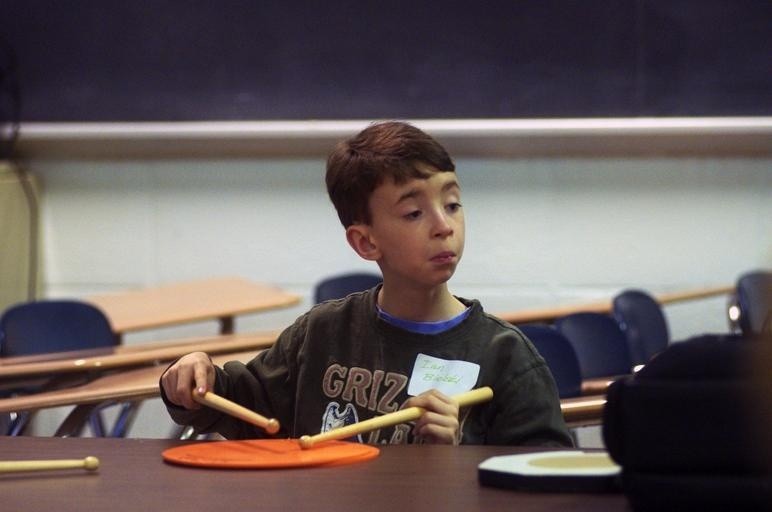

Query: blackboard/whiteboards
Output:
[0,0,772,162]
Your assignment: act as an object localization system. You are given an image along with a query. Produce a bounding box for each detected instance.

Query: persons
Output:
[150,117,577,449]
